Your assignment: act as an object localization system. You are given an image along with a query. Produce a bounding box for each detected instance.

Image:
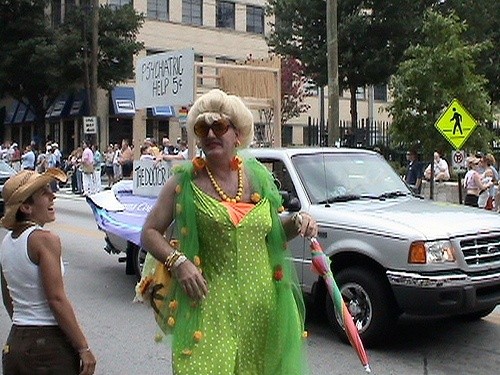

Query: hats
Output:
[51,143,59,147]
[0,168,68,229]
[468,157,481,163]
[11,143,18,148]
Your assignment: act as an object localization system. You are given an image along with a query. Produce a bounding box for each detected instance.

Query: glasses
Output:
[406,153,410,155]
[194,119,232,138]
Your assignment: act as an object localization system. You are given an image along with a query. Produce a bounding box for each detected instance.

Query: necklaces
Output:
[204,155,244,204]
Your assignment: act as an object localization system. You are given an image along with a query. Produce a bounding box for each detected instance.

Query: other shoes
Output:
[75,189,86,197]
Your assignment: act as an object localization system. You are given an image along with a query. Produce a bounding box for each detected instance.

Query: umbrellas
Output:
[293,213,371,374]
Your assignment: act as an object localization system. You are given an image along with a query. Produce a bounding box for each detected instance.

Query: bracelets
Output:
[164,249,187,273]
[78,345,91,353]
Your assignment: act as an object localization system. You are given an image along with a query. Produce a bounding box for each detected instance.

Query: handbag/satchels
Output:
[150,261,182,335]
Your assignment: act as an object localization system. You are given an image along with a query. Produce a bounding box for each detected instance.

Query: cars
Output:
[0,160,18,203]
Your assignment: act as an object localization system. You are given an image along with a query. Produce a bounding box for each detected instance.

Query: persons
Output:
[463,151,500,211]
[140,89,319,375]
[403,149,424,195]
[0,166,96,375]
[0,134,207,198]
[423,152,451,183]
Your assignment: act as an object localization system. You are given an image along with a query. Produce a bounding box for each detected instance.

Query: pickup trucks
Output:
[104,146,499,344]
